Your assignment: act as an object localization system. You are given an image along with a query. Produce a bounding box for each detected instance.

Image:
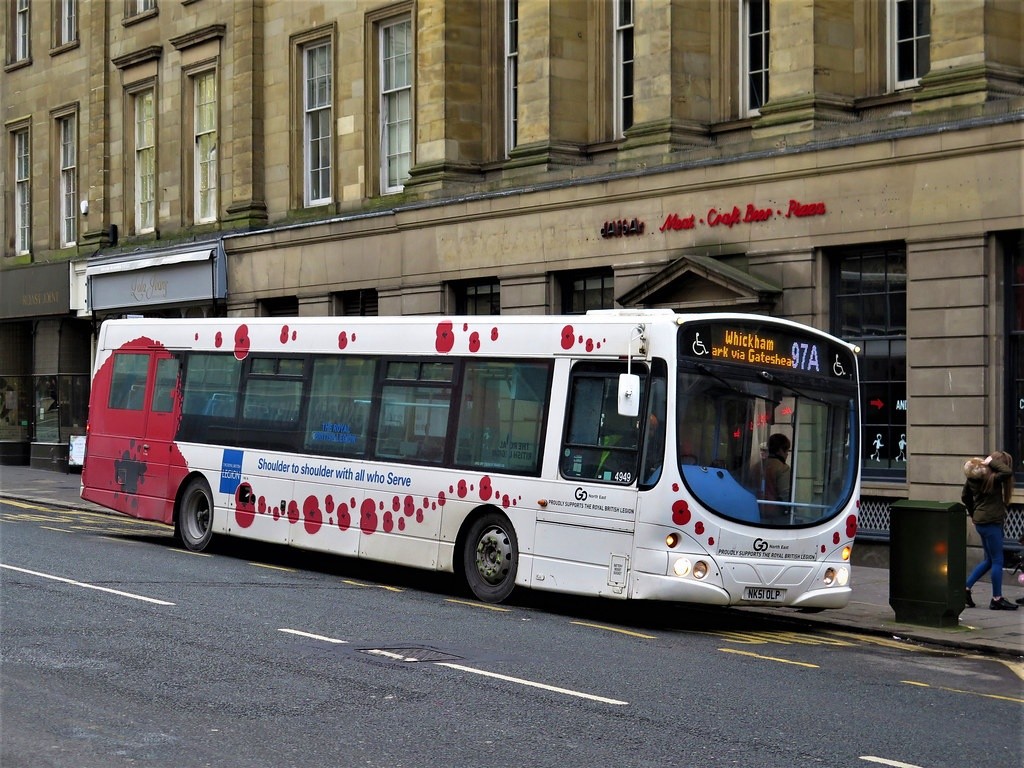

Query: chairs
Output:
[125,384,470,464]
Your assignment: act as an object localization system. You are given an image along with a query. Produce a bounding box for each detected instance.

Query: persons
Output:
[594,413,658,488]
[957,450,1019,611]
[753,432,793,518]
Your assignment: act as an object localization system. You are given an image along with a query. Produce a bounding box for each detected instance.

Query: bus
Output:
[78,306,862,609]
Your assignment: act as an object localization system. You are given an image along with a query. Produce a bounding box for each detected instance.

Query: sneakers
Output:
[963,590,976,608]
[989,597,1018,610]
[1015,597,1024,605]
